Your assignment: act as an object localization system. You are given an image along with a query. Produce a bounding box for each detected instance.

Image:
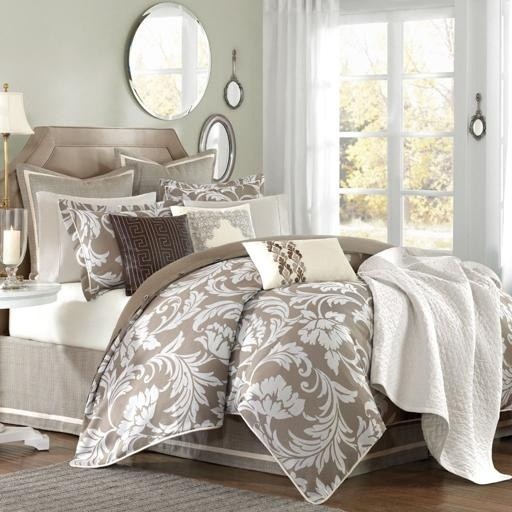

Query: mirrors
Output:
[126,0,213,120]
[467,93,486,140]
[197,113,237,183]
[223,49,244,108]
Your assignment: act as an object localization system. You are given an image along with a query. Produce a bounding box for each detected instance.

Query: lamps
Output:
[0,82,35,206]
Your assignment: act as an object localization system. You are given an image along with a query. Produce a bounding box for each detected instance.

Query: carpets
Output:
[0,457,341,512]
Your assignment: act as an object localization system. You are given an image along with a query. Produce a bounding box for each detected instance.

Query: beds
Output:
[0,125,512,505]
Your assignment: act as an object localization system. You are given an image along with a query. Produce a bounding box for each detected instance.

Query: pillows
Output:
[14,148,292,302]
[240,238,358,291]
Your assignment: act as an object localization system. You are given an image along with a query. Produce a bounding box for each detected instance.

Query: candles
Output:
[3,225,21,265]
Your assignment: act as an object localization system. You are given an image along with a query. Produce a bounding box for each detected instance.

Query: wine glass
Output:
[0,207,30,291]
